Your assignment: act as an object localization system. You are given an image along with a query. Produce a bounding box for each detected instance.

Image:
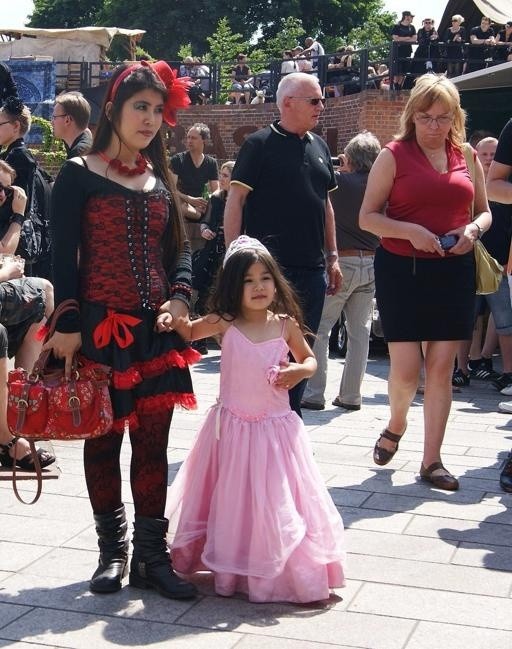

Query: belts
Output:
[337,249,376,257]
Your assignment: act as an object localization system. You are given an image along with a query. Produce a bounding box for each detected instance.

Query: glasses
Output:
[413,110,454,125]
[289,95,325,106]
[0,182,13,198]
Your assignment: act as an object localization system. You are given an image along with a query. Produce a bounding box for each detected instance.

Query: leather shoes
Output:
[420,461,460,491]
[373,421,407,466]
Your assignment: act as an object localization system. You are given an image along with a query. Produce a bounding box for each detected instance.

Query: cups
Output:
[0,253,26,272]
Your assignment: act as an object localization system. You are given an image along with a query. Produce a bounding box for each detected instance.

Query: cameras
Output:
[331,157,345,168]
[438,233,460,249]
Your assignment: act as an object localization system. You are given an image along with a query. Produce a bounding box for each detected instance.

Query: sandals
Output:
[0,437,56,470]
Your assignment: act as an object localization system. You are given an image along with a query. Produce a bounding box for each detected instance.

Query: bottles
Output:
[202,183,209,201]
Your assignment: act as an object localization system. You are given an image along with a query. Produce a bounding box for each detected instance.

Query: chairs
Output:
[179,56,381,103]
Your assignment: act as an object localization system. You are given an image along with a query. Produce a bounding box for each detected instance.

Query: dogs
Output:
[250,88,267,104]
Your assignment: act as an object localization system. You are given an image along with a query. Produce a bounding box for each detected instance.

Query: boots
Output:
[89,505,130,592]
[131,512,199,601]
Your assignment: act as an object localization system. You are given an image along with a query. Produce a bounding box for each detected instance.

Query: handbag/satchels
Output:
[474,238,504,296]
[180,200,201,221]
[6,366,113,441]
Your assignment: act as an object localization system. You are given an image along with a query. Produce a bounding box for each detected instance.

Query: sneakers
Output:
[332,396,360,410]
[500,454,512,493]
[453,358,512,413]
[300,400,325,409]
